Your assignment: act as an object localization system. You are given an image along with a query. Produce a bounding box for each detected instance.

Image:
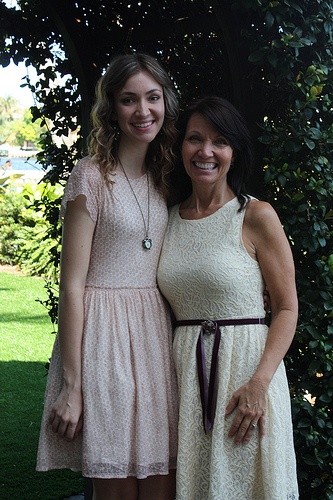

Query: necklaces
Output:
[117,154,152,250]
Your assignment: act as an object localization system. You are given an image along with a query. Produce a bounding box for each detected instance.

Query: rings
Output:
[250,423,258,429]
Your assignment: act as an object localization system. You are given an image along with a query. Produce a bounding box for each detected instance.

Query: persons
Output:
[36,54,179,500]
[156,93,300,500]
